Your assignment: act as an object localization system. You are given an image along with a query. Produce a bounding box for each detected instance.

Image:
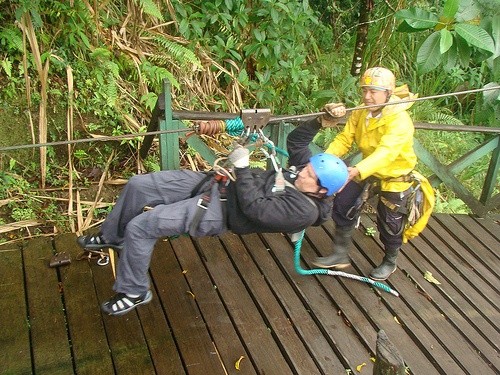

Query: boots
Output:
[369,246,400,281]
[311,225,352,269]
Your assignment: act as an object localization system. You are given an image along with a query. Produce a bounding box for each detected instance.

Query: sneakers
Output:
[77,232,126,250]
[101,289,153,317]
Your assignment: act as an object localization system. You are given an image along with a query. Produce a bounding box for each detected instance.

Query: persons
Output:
[311,66,419,281]
[77,102,348,315]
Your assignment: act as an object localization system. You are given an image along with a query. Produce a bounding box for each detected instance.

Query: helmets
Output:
[310,152,349,197]
[359,67,397,92]
